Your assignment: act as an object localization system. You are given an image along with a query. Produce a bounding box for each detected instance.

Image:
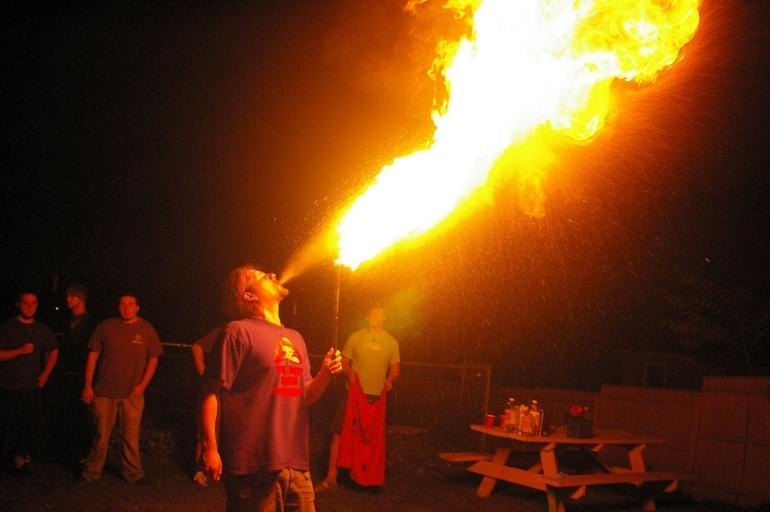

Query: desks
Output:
[468,422,669,512]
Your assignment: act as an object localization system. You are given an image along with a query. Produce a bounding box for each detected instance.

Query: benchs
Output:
[437,448,687,512]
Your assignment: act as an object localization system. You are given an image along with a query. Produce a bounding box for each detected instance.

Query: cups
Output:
[499,415,504,428]
[481,414,496,429]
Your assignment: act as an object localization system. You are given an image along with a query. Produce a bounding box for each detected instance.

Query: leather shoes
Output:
[136,479,152,485]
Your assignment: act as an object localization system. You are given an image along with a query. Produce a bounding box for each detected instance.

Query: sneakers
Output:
[15,454,35,477]
[314,478,338,492]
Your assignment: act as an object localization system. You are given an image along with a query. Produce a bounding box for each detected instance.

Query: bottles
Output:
[504,398,547,439]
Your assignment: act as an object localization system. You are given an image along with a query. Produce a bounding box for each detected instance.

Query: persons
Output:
[0,285,163,485]
[315,301,401,493]
[192,267,343,512]
